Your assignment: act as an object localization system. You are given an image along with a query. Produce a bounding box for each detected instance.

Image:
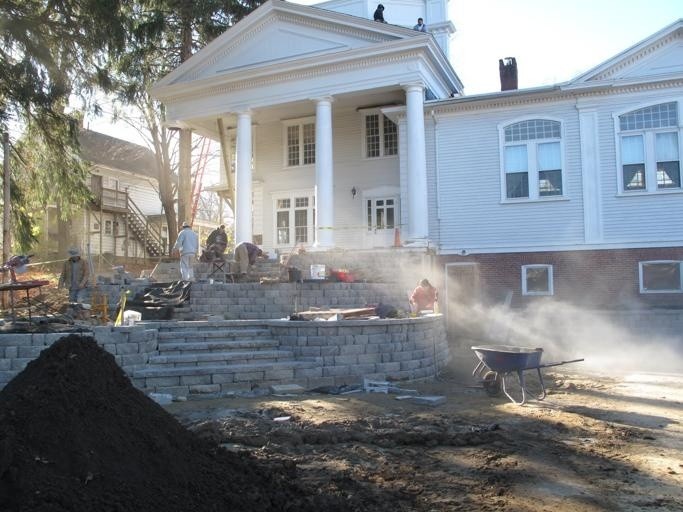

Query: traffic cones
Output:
[390,228,404,248]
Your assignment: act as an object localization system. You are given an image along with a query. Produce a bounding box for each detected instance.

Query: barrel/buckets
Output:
[310,264,325,279]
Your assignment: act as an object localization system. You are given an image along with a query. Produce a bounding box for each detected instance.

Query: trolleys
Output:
[471,344,584,406]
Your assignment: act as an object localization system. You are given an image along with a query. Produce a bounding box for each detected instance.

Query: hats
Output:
[182,222,191,226]
[70,248,81,256]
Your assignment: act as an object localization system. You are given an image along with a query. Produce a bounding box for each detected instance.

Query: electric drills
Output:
[0,254,35,282]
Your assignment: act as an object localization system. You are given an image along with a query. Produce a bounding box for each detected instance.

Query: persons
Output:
[232,242,263,279]
[57,248,92,304]
[374,3,386,24]
[204,225,227,255]
[409,278,441,318]
[170,220,199,283]
[413,18,425,34]
[286,248,308,276]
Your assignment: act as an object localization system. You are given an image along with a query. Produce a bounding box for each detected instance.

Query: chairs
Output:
[91,292,109,323]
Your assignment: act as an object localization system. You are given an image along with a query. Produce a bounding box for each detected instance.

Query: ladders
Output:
[190,136,213,229]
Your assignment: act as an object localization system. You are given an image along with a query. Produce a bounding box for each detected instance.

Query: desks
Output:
[0,280,50,325]
